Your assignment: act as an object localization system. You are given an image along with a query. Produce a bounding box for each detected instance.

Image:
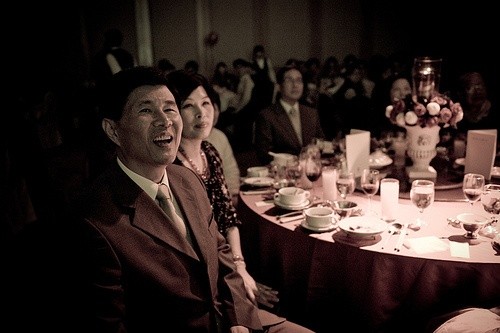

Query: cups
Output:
[273,153,294,164]
[322,166,337,202]
[274,187,311,205]
[305,207,335,227]
[247,166,270,178]
[380,178,399,221]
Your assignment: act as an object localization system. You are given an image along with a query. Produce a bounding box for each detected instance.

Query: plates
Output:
[274,198,310,210]
[490,167,500,178]
[300,219,339,231]
[271,159,298,166]
[244,176,274,186]
[369,159,393,169]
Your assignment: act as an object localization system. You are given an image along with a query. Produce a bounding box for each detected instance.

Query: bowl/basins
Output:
[339,217,389,237]
[330,200,360,216]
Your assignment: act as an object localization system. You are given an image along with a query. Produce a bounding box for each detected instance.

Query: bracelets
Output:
[233,257,246,265]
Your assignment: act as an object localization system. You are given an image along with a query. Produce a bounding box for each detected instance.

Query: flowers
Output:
[385,94,463,128]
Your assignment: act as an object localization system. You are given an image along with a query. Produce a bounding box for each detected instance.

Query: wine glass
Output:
[463,173,484,213]
[336,168,356,200]
[410,180,435,230]
[304,158,322,199]
[286,159,304,188]
[480,183,500,234]
[361,169,379,215]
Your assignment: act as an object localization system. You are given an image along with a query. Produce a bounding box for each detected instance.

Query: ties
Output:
[290,107,303,146]
[155,183,195,252]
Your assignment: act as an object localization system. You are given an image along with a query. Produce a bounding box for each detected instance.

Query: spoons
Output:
[381,222,401,249]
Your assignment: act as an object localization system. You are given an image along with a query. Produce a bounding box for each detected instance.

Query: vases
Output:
[405,126,442,184]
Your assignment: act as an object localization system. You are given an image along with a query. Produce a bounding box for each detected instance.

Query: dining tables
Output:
[239,165,500,333]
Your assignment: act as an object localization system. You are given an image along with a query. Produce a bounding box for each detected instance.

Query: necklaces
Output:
[178,145,210,183]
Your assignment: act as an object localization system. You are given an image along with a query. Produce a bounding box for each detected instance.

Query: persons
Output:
[57,30,500,333]
